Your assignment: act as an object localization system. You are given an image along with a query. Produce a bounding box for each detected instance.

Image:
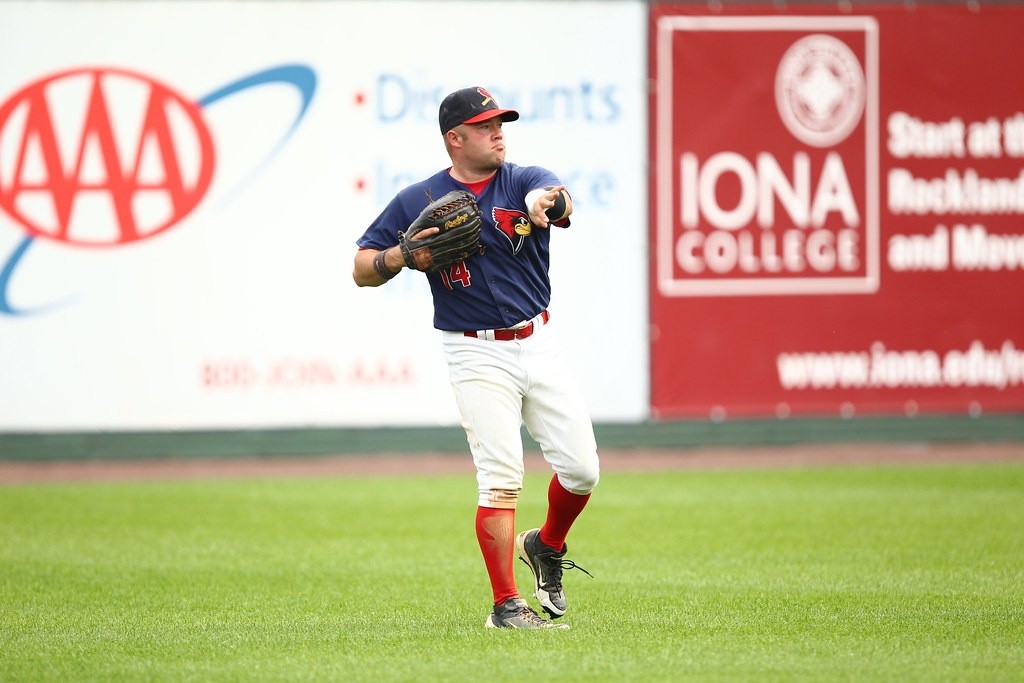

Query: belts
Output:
[463,310,550,342]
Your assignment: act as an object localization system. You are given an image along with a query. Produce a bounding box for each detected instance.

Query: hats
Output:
[439,87,520,135]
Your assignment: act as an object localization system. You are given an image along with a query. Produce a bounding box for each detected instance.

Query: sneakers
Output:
[516,528,595,617]
[484,594,569,632]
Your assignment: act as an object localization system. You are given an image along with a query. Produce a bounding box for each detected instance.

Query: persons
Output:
[353,88,600,633]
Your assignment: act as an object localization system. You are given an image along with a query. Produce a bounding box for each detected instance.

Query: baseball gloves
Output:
[397,188,487,273]
